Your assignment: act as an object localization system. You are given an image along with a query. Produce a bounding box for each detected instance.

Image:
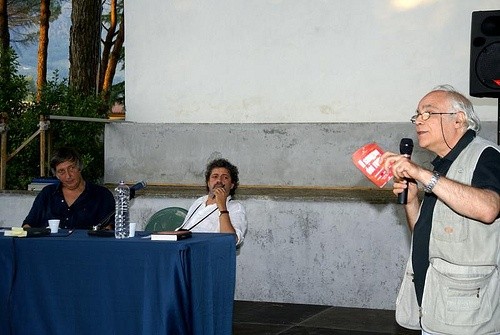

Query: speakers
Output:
[469,10,500,99]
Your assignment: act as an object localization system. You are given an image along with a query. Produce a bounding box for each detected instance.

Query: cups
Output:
[48,219,60,234]
[128,223,136,238]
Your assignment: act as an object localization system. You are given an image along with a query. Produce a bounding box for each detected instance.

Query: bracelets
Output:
[220,211,229,213]
[424,170,440,194]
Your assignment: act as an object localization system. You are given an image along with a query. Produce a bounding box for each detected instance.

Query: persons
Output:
[175,159,248,249]
[21,146,116,232]
[378,84,500,335]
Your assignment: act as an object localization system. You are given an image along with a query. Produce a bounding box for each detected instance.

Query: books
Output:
[150,231,193,242]
[4,227,52,237]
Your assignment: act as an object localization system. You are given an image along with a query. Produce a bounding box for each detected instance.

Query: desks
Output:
[0,229,237,335]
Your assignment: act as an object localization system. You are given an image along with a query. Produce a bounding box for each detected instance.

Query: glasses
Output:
[410,112,456,124]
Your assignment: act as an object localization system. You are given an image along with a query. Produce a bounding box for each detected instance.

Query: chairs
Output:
[144,207,188,233]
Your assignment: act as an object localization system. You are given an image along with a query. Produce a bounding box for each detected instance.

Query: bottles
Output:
[112,180,130,240]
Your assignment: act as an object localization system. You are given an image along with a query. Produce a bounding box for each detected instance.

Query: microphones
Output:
[397,138,413,204]
[130,181,146,192]
